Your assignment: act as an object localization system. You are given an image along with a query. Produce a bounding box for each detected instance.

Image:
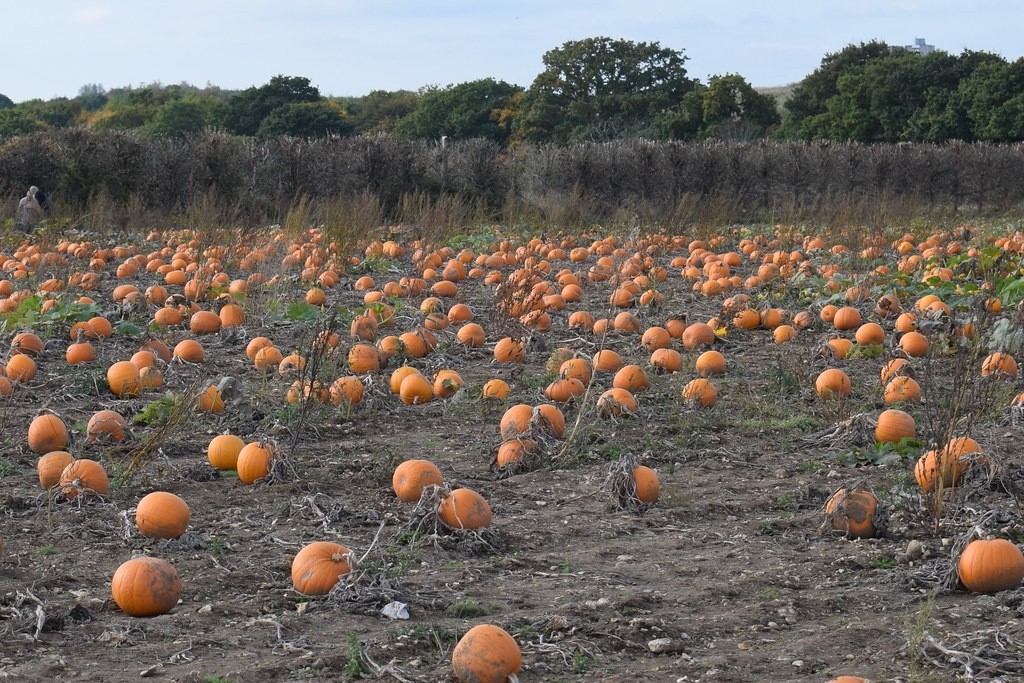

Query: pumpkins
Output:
[827,676,873,683]
[453,625,521,683]
[0,219,1024,615]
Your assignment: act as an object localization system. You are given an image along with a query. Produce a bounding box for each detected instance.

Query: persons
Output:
[18,187,50,230]
